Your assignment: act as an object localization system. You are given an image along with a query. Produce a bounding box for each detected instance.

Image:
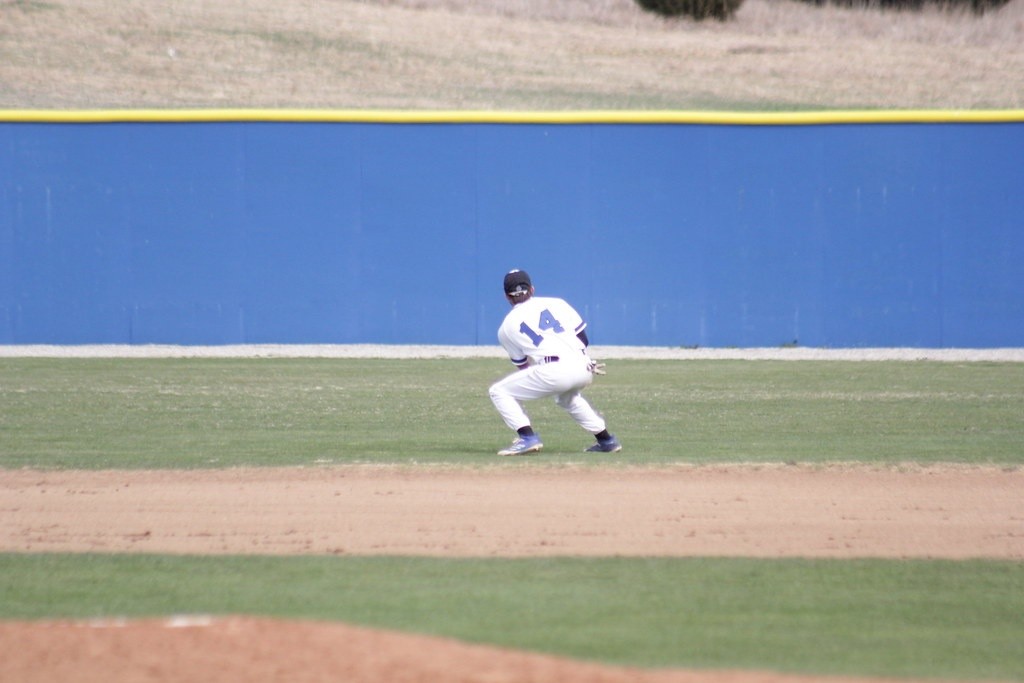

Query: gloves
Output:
[587,360,606,376]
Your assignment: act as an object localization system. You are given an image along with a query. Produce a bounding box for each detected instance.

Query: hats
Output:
[504,269,532,298]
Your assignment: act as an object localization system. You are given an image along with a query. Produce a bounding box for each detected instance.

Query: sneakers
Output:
[498,433,542,457]
[584,434,622,454]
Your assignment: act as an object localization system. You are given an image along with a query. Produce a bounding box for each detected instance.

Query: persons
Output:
[486,268,626,458]
